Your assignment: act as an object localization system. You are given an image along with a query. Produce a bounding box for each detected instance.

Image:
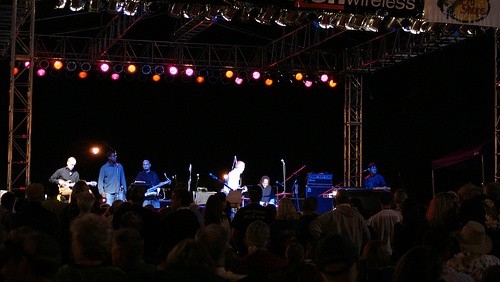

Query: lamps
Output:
[170,0,301,27]
[318,12,433,35]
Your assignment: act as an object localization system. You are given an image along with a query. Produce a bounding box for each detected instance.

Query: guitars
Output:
[57,179,98,194]
[144,178,172,197]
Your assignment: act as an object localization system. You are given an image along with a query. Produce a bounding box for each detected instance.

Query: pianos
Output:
[333,187,391,190]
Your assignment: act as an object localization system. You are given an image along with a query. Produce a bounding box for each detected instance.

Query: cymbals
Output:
[274,192,293,196]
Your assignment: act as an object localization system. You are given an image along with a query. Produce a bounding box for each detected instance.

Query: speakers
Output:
[306,185,334,214]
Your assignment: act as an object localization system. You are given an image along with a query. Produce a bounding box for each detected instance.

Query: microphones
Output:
[276,181,284,187]
[364,168,370,172]
[189,164,192,171]
[235,156,237,165]
[197,174,199,179]
[281,159,285,165]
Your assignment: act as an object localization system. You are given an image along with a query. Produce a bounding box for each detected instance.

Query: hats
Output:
[315,233,358,276]
[453,220,493,253]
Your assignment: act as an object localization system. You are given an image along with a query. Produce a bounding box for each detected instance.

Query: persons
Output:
[222,161,245,219]
[133,159,161,209]
[257,175,272,206]
[98,151,128,206]
[0,181,500,282]
[364,163,385,189]
[50,157,81,203]
